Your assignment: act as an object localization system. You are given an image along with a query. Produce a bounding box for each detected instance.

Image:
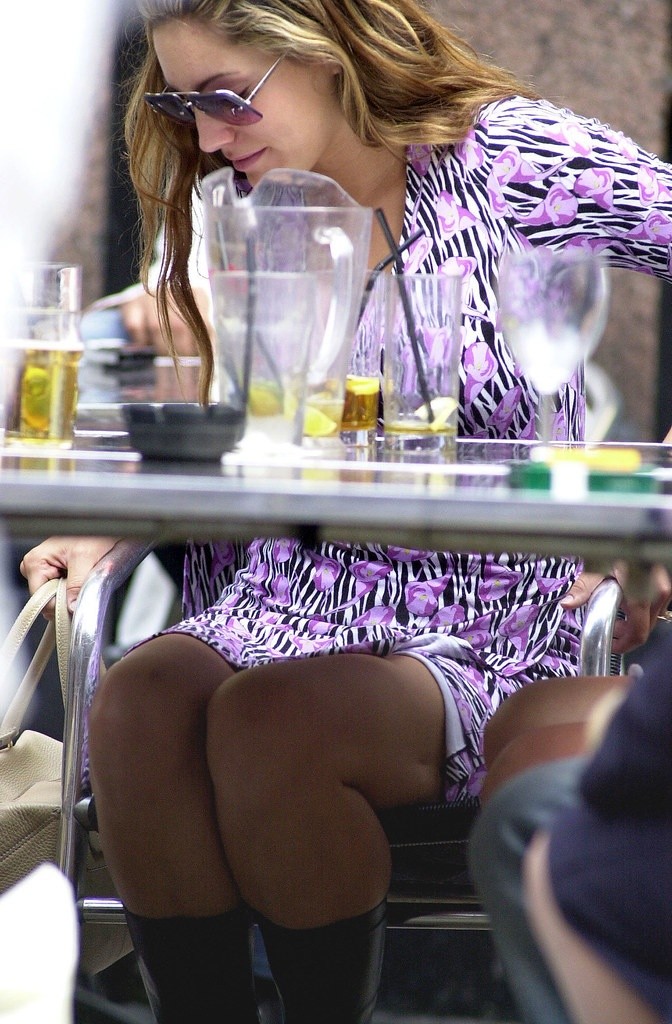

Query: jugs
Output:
[201,167,372,466]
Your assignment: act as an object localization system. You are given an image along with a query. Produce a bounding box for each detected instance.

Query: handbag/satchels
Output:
[0,577,135,979]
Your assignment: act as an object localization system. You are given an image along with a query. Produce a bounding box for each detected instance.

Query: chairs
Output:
[56,538,627,1024]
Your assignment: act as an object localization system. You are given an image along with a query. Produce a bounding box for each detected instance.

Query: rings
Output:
[657,611,672,623]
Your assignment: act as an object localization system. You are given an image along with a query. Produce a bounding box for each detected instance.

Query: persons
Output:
[19,0,672,1024]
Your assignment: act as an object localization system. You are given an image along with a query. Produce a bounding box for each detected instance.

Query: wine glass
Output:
[500,245,609,458]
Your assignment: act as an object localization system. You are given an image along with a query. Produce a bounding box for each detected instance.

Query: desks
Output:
[0,431,672,603]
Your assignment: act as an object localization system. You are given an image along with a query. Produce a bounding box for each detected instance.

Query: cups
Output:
[212,271,312,461]
[0,261,83,452]
[313,270,387,431]
[383,275,462,459]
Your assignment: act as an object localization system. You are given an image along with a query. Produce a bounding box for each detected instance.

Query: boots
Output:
[251,899,387,1024]
[122,906,258,1024]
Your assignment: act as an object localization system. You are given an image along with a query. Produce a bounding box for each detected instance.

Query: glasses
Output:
[143,49,288,128]
